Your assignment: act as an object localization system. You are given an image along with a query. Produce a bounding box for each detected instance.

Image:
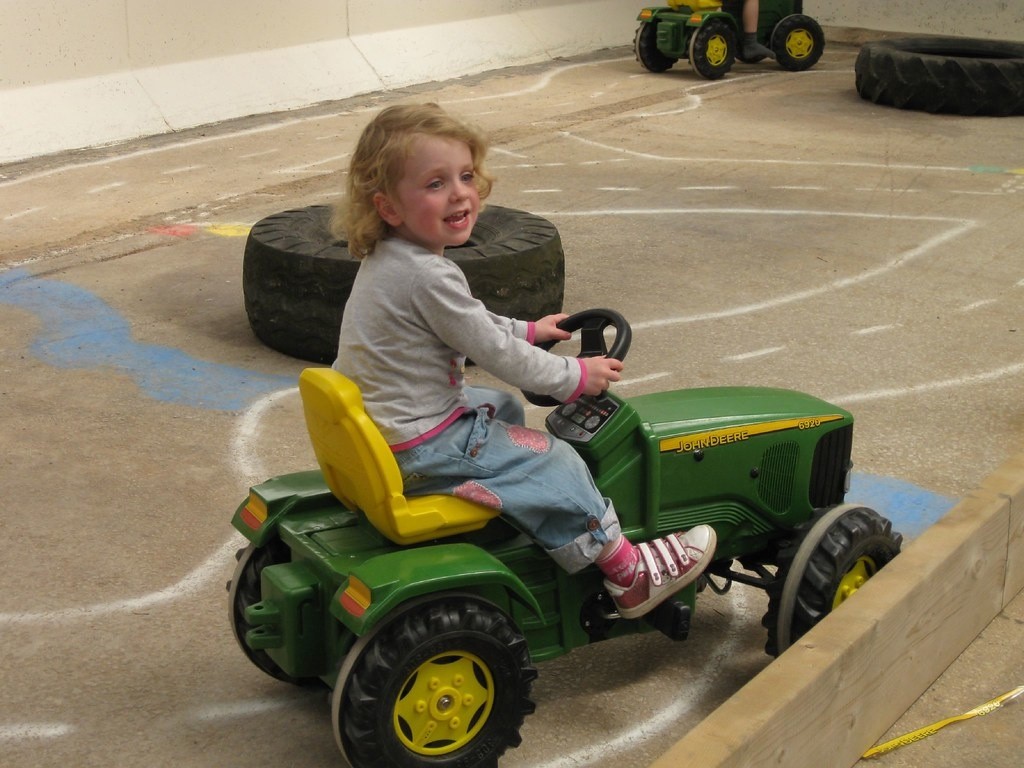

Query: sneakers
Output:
[603,523,717,620]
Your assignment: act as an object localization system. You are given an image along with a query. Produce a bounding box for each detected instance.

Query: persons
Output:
[328,102,718,620]
